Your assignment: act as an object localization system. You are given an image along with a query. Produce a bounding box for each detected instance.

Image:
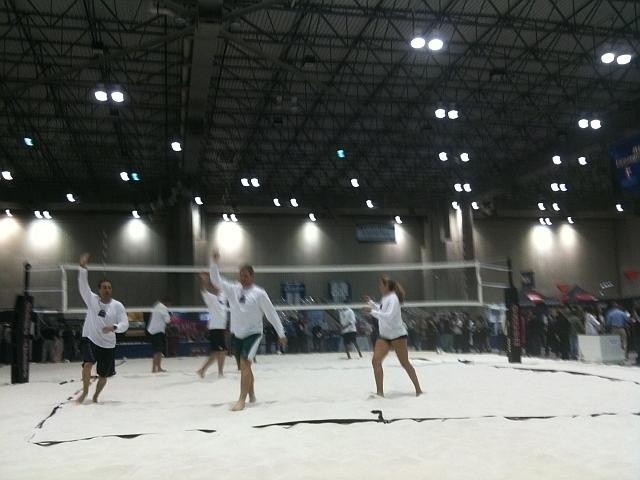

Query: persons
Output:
[209,248,288,411]
[365,276,423,398]
[196,272,228,378]
[77,252,130,403]
[339,306,363,359]
[0,319,84,362]
[521,301,640,365]
[406,310,491,353]
[263,316,335,353]
[146,295,173,373]
